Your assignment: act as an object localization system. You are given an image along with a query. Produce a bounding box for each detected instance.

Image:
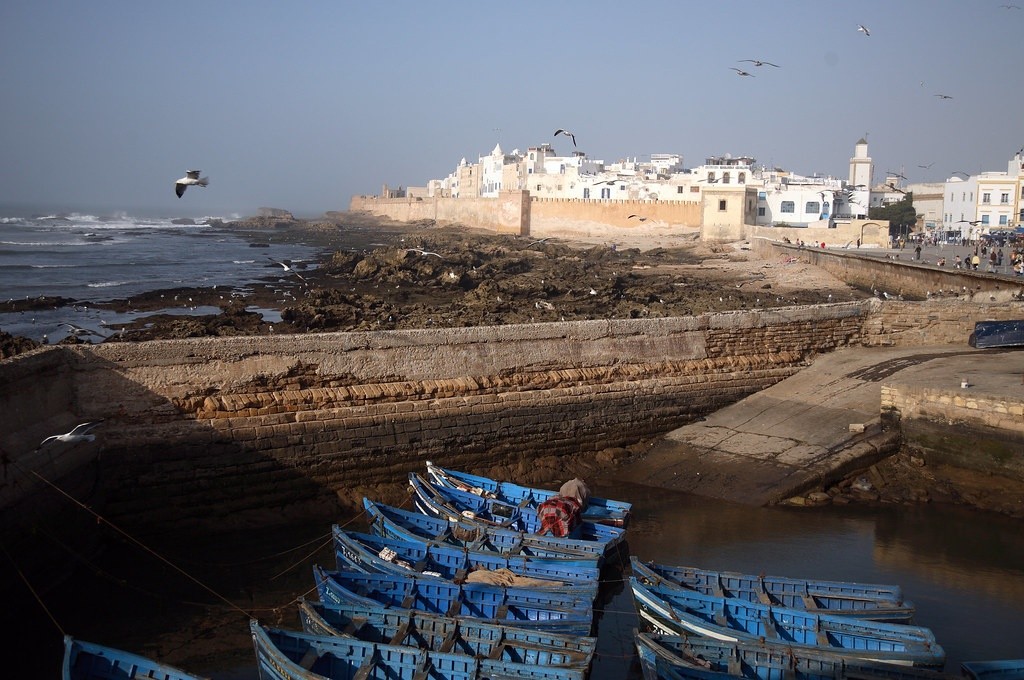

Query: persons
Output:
[980,246,1003,273]
[783,237,825,249]
[921,234,937,250]
[957,236,970,247]
[1010,248,1024,277]
[915,245,921,260]
[885,253,890,258]
[972,253,980,270]
[964,254,971,270]
[937,257,946,267]
[857,238,860,248]
[953,255,962,268]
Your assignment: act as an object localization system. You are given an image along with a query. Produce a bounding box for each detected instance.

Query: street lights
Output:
[948,224,961,260]
[969,226,984,254]
[1000,226,1017,274]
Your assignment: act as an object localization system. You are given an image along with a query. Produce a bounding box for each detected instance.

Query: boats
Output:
[249,460,633,680]
[964,659,1024,680]
[62,635,202,680]
[626,553,947,680]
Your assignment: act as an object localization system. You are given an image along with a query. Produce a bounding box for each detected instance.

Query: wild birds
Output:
[729,67,756,78]
[856,23,871,37]
[738,59,780,68]
[174,169,210,198]
[998,5,1021,10]
[816,184,867,196]
[34,418,104,450]
[883,171,911,194]
[553,129,577,147]
[952,170,970,177]
[917,162,936,169]
[954,220,985,226]
[933,94,952,100]
